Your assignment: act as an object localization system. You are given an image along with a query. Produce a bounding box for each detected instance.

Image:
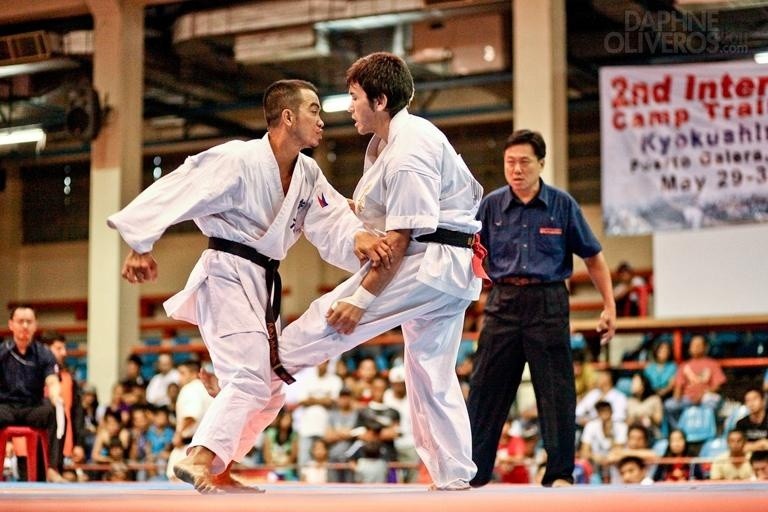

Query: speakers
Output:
[62,86,101,141]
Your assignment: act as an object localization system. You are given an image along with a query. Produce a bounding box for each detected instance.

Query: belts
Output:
[495,275,545,287]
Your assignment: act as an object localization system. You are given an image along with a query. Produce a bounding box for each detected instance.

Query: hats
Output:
[389,365,406,384]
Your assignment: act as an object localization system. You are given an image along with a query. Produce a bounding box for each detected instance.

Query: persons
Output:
[198,52,485,491]
[106,77,399,497]
[465,126,618,486]
[1,262,768,483]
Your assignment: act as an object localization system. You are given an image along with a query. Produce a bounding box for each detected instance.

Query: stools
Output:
[0,424,50,481]
